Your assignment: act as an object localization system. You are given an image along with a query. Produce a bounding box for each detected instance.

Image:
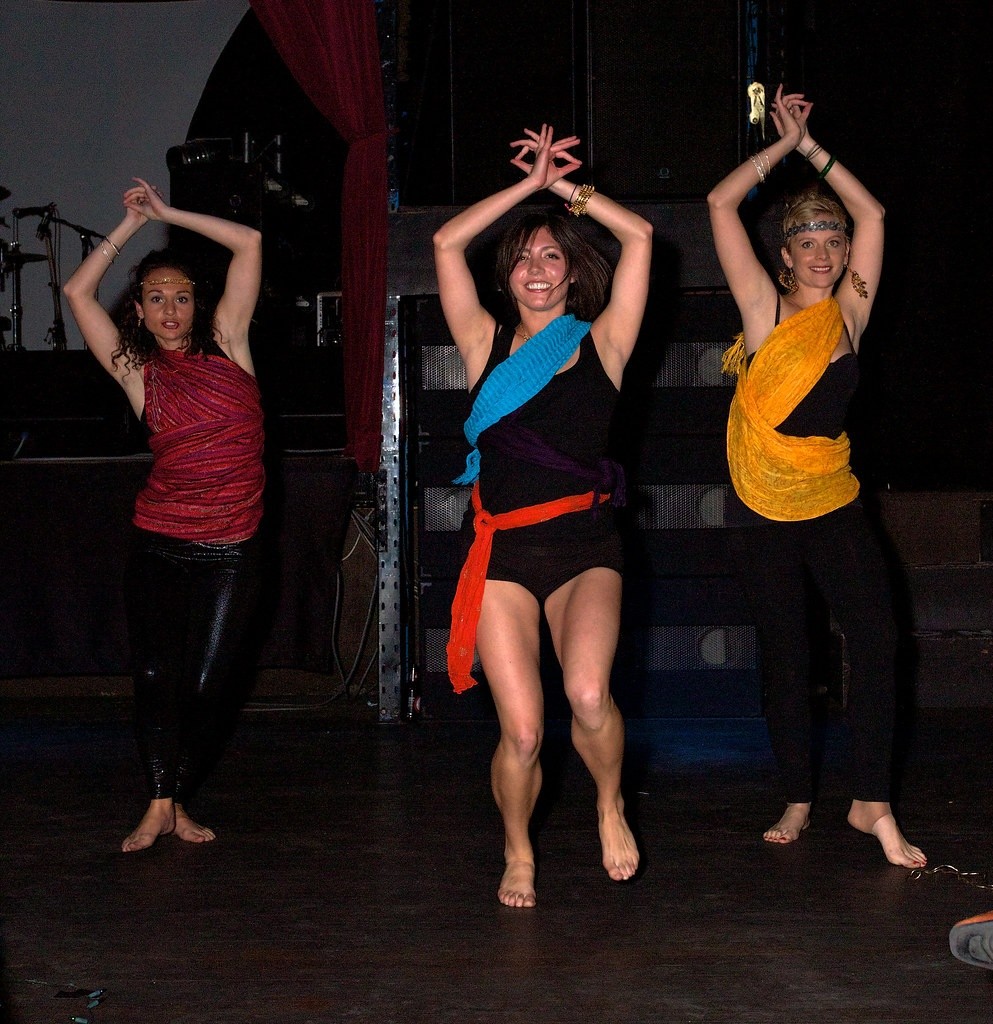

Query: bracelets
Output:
[99,235,120,265]
[565,183,595,217]
[805,143,823,160]
[817,154,836,178]
[747,148,771,184]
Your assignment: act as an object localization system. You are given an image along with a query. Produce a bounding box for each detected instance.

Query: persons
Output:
[61,176,266,854]
[948,910,993,971]
[432,124,654,908]
[707,82,929,868]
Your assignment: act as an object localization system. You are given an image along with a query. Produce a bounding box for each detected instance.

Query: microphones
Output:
[35,204,54,239]
[12,206,51,219]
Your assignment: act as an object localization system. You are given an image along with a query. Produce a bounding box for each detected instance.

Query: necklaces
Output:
[516,323,532,342]
